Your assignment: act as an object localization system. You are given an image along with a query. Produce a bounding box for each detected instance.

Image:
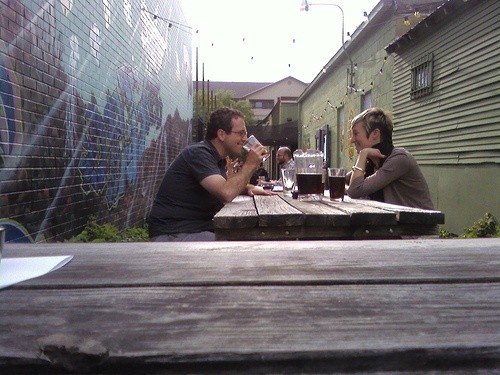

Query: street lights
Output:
[300,0,353,94]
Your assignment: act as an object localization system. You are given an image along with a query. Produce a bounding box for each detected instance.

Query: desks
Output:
[213,185,445,241]
[0,238,500,375]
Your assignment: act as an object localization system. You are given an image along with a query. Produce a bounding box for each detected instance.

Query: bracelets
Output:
[352,166,365,174]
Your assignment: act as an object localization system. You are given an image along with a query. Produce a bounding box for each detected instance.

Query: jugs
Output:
[293,149,324,201]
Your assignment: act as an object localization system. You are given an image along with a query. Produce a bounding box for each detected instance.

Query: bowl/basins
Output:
[263,184,274,191]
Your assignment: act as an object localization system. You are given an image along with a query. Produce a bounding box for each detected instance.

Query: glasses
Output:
[230,130,248,137]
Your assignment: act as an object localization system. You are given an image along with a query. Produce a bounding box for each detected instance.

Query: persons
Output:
[225,147,295,192]
[148,107,277,242]
[345,107,439,239]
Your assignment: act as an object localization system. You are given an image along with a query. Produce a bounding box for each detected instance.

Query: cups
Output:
[327,168,346,202]
[242,134,271,163]
[281,168,295,197]
[259,176,265,186]
[321,169,326,196]
[291,186,298,199]
